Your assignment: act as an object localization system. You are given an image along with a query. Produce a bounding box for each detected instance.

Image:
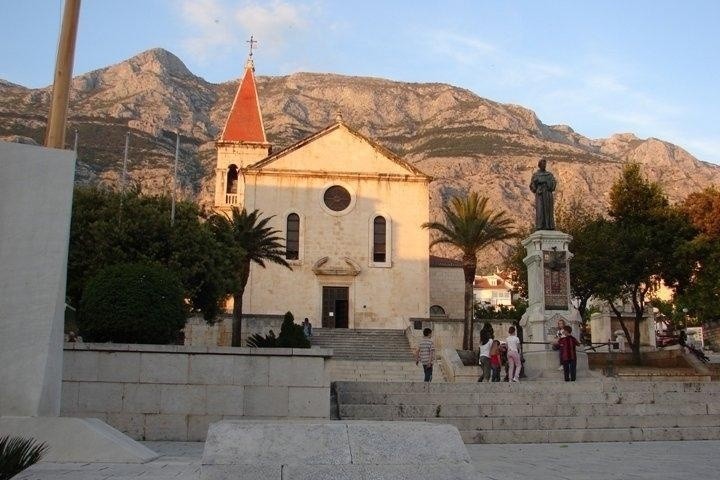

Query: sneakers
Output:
[558,365,563,370]
[509,377,520,382]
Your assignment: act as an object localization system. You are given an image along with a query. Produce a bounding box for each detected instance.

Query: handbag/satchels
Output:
[489,341,498,356]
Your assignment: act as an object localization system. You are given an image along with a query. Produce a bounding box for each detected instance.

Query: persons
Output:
[301,318,313,338]
[67,331,78,342]
[554,324,580,381]
[552,319,571,370]
[476,331,494,382]
[529,158,557,230]
[489,339,503,382]
[415,328,437,383]
[504,326,524,383]
